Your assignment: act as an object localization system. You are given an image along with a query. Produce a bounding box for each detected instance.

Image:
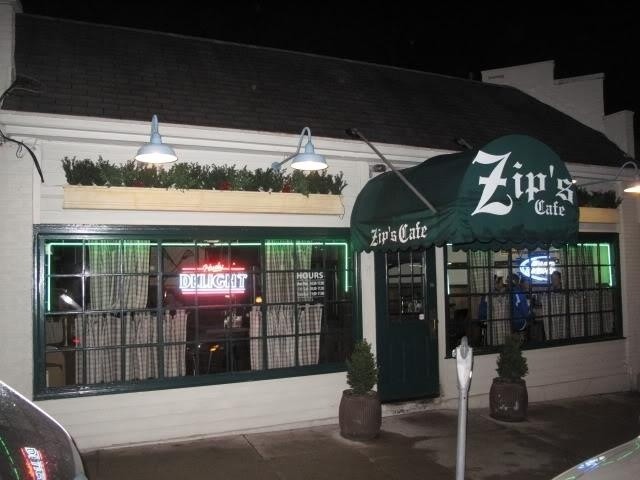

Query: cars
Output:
[551,436,639,480]
[0,381,87,480]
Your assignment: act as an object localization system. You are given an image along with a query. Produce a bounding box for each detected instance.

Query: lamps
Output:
[576,162,640,193]
[135,114,178,164]
[272,126,329,170]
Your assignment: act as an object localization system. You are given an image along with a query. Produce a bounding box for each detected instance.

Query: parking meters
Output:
[449,331,476,480]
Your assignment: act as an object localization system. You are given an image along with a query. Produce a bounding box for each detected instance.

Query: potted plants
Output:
[339,338,382,441]
[489,334,530,422]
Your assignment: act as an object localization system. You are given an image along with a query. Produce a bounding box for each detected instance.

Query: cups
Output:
[223,310,232,329]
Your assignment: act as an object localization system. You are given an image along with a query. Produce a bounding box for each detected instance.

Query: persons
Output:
[477,270,562,333]
